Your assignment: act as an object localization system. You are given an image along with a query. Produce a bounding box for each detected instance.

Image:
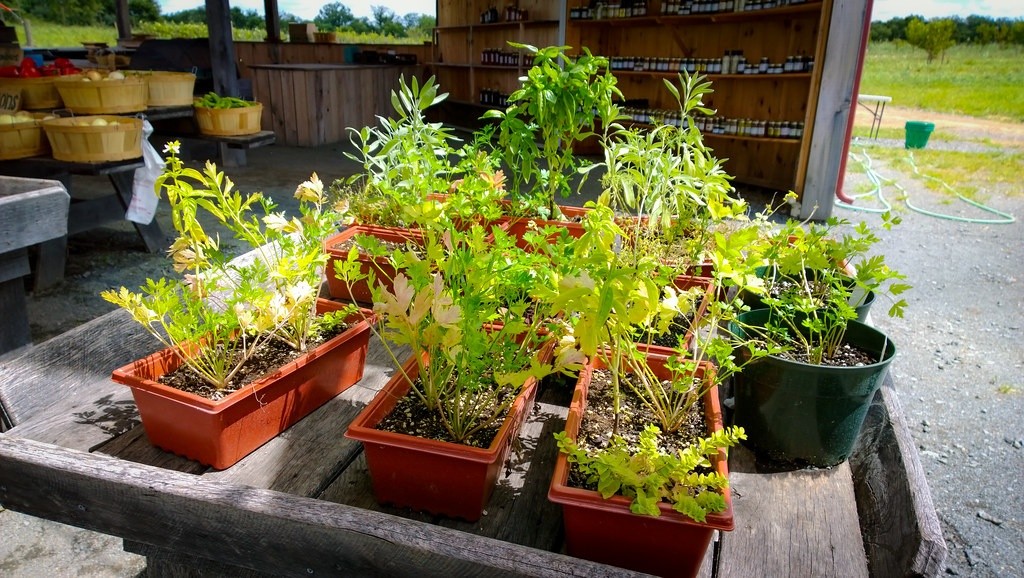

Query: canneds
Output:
[479,0,815,139]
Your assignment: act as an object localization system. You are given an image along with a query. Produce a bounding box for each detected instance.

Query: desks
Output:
[857,94,893,139]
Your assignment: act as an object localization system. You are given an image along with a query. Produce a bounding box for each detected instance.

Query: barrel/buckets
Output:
[905,121,935,149]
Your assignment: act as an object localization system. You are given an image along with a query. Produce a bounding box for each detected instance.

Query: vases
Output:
[342,321,556,523]
[112,296,375,471]
[737,264,876,324]
[450,200,590,254]
[100,38,914,525]
[598,272,718,359]
[726,306,897,468]
[321,225,430,305]
[547,347,736,578]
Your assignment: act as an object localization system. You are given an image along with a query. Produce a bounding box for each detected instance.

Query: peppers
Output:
[0,57,83,79]
[193,91,258,109]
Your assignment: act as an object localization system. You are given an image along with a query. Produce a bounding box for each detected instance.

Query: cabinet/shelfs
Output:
[428,0,562,113]
[563,0,833,204]
